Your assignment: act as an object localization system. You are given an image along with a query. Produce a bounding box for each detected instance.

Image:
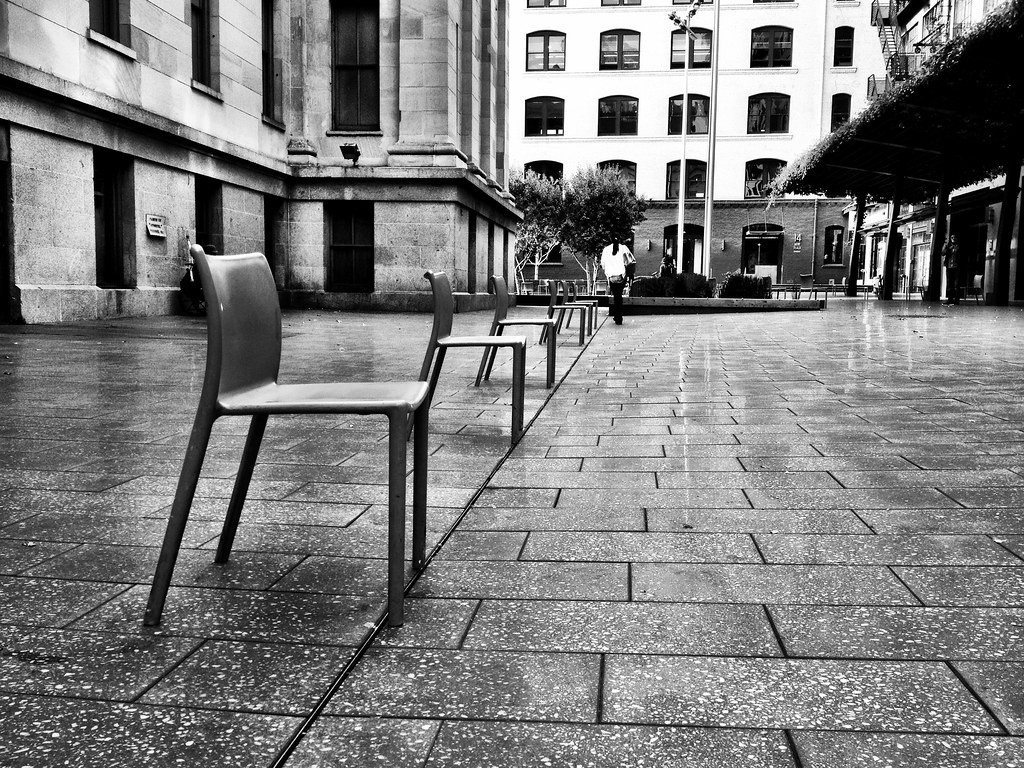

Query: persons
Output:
[600,232,627,325]
[660,254,675,277]
[943,233,965,306]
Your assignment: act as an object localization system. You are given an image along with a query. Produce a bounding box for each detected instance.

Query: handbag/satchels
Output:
[623,252,637,275]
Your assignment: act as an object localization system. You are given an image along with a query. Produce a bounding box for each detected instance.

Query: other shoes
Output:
[942,300,954,306]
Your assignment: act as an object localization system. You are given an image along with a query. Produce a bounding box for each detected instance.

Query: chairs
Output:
[566,282,598,329]
[832,276,847,297]
[555,280,593,337]
[797,274,828,310]
[905,275,925,302]
[406,270,527,445]
[539,280,586,346]
[474,275,558,390]
[857,287,869,300]
[960,274,986,306]
[708,277,723,298]
[142,245,425,628]
[762,276,787,299]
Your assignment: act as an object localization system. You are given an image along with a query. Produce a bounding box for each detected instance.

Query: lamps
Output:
[339,142,361,167]
